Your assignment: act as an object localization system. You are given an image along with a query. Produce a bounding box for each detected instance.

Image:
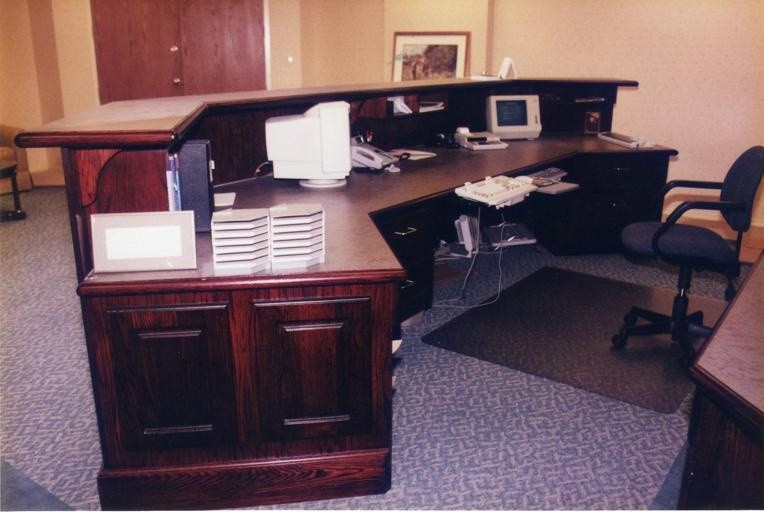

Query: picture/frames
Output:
[391,31,471,83]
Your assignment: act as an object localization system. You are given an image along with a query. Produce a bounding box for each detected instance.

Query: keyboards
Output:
[455,174,538,210]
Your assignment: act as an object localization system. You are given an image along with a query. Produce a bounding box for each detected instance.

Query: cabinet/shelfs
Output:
[370,205,435,322]
[561,152,669,255]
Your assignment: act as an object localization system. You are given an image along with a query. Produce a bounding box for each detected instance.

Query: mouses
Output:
[515,175,532,183]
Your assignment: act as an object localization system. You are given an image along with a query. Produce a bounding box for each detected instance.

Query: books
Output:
[418,101,446,113]
[484,220,538,248]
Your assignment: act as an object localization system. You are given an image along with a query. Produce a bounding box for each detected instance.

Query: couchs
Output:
[0,125,33,195]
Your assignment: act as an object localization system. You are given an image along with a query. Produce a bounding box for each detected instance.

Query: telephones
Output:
[350,135,400,173]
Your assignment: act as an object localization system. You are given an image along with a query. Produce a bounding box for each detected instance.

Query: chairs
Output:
[611,145,764,368]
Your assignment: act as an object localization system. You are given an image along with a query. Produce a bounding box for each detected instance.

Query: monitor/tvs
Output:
[486,93,542,140]
[264,100,351,188]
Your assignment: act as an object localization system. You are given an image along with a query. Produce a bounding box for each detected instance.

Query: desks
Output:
[0,160,21,211]
[677,250,764,510]
[14,78,679,511]
